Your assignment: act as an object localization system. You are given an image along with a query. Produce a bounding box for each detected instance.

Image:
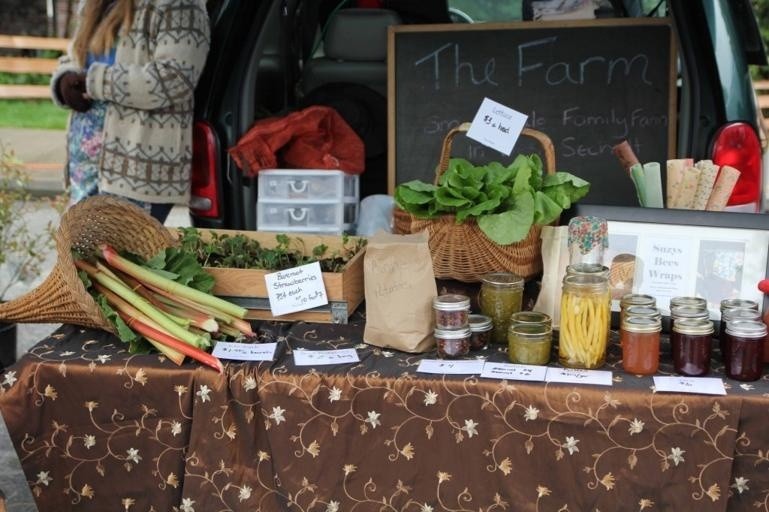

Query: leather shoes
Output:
[57,69,93,115]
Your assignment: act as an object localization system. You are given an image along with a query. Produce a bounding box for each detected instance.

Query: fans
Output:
[386,17,679,209]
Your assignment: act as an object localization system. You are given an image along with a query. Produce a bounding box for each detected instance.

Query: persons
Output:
[48,0,213,226]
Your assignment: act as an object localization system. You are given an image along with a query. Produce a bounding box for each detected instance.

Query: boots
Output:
[184,0,769,242]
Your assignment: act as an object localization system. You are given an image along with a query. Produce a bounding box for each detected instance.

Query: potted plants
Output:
[572,203,768,336]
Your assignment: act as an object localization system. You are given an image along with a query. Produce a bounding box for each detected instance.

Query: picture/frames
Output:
[21,324,767,510]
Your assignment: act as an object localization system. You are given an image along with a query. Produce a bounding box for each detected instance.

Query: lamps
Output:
[306,6,406,100]
